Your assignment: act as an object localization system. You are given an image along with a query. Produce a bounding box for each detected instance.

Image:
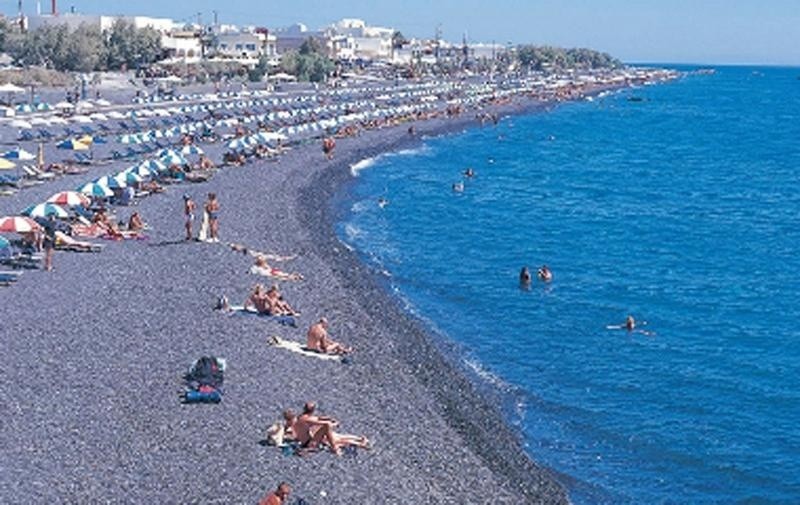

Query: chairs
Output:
[21,164,58,183]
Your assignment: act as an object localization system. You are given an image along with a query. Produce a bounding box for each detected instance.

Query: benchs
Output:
[0,271,24,286]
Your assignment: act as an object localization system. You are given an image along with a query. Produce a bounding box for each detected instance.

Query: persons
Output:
[475,82,587,103]
[211,107,358,167]
[600,315,649,336]
[291,402,344,457]
[280,406,367,450]
[305,315,354,354]
[17,179,219,274]
[368,95,467,130]
[172,132,217,183]
[258,481,292,505]
[516,266,534,294]
[210,283,301,320]
